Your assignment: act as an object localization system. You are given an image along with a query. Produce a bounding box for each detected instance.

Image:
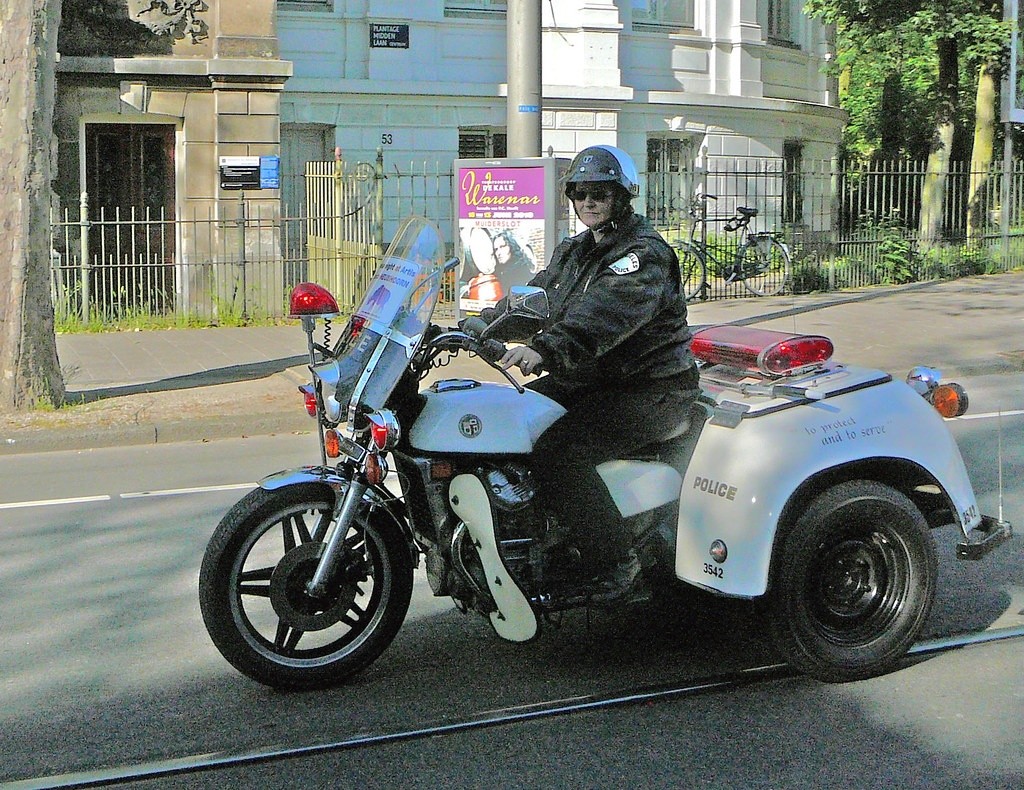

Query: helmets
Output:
[565,144,640,202]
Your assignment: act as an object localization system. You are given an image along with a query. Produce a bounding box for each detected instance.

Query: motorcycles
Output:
[192,213,1015,682]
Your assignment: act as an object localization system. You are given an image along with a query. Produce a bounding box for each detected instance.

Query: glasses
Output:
[570,189,614,202]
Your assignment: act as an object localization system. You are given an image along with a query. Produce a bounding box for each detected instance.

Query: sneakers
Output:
[586,555,653,604]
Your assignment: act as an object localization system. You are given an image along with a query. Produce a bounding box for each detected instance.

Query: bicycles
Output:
[657,192,793,301]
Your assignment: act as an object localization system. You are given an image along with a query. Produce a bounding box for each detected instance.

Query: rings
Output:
[522,359,528,365]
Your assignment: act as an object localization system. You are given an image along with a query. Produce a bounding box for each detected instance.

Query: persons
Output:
[492,231,535,301]
[396,144,701,606]
